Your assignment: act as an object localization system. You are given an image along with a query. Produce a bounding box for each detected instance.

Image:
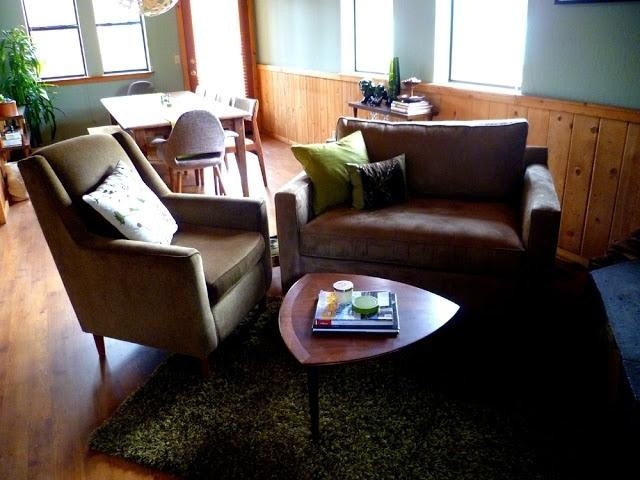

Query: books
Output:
[311,289,400,334]
[391,99,434,116]
[0,125,24,148]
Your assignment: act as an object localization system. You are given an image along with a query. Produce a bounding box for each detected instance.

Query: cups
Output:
[333,279,354,306]
[161,94,171,107]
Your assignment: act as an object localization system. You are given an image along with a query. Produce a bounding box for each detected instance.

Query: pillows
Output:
[81,160,178,247]
[290,130,371,219]
[343,153,412,212]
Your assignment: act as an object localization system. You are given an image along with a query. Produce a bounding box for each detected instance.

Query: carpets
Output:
[588,262,639,401]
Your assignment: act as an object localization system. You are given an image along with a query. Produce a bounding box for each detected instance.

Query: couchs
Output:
[274,116,561,297]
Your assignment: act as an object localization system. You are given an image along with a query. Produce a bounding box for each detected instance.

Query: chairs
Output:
[17,131,272,376]
[126,81,267,188]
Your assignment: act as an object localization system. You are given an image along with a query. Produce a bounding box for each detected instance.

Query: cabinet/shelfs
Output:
[0,105,31,159]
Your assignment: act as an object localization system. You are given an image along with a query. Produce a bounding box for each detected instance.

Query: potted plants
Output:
[0,93,18,117]
[0,24,66,147]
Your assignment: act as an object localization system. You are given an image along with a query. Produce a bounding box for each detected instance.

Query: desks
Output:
[348,101,440,120]
[99,89,253,196]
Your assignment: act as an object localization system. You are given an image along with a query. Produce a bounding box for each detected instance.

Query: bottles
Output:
[386,56,401,106]
